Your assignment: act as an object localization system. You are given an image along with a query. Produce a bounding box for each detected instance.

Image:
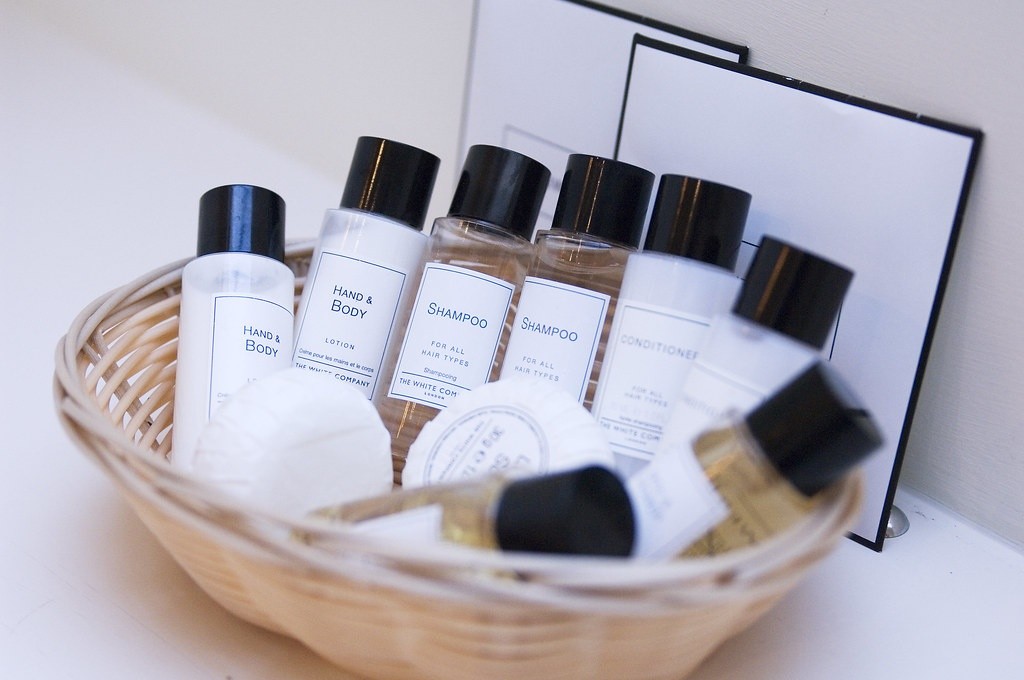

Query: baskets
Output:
[46,229,864,680]
